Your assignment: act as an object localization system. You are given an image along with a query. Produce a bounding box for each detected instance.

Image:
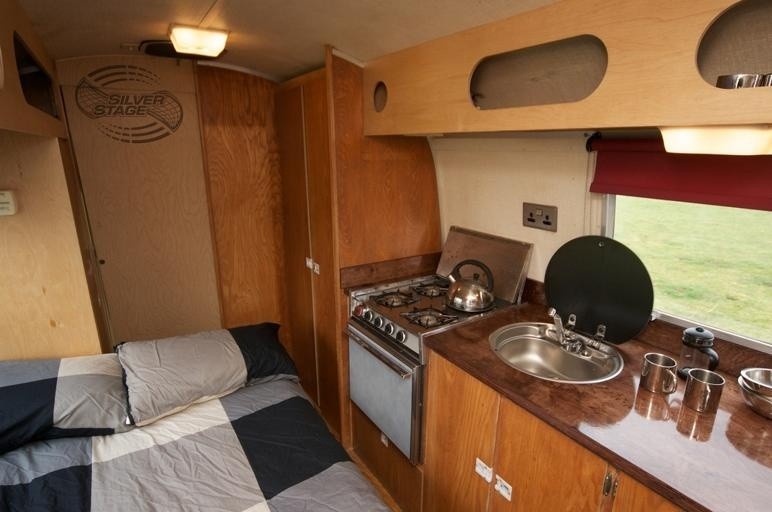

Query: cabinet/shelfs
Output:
[349,404,423,512]
[600,457,684,512]
[426,350,607,508]
[273,55,441,440]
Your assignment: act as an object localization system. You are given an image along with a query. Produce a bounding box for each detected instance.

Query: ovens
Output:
[348,315,424,464]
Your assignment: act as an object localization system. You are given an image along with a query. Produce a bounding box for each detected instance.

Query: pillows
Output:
[114,321,300,428]
[0,351,131,457]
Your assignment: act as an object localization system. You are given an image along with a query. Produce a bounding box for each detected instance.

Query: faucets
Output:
[547,307,585,353]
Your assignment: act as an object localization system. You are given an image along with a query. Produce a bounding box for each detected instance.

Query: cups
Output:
[682,367,726,414]
[677,326,720,382]
[640,352,678,395]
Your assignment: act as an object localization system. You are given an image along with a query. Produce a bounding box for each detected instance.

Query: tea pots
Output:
[445,259,496,315]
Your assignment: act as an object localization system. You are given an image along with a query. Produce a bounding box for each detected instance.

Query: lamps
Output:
[166,24,232,57]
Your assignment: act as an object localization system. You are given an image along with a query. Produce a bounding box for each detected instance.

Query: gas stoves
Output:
[349,275,534,358]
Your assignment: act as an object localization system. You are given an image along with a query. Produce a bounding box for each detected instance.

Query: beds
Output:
[0,380,395,512]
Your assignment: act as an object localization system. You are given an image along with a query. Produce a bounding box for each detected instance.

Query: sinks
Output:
[486,319,626,385]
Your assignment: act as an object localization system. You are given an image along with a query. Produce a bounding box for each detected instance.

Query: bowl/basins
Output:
[737,367,772,419]
[763,73,772,86]
[715,74,763,89]
